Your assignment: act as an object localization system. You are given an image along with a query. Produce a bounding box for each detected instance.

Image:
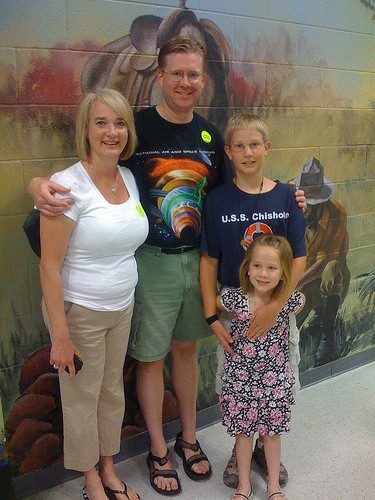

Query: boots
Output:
[314,293,341,363]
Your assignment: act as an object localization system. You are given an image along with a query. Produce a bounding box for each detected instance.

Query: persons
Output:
[26,36,307,496]
[216,235,306,500]
[200,112,307,487]
[39,87,149,499]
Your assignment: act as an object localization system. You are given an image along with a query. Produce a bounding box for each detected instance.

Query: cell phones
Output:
[65,354,83,375]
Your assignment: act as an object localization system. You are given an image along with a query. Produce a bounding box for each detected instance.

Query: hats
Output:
[294,157,334,204]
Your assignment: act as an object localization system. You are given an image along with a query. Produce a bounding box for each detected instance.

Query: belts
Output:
[161,246,199,254]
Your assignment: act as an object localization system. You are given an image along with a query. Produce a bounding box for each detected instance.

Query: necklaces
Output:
[234,180,263,246]
[87,162,119,195]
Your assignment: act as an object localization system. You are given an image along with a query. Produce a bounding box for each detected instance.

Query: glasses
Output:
[160,69,202,83]
[226,142,265,153]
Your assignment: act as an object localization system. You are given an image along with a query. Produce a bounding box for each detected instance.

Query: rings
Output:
[34,205,37,210]
[54,364,59,369]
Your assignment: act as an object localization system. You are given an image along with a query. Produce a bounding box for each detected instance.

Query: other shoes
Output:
[253,438,288,483]
[232,485,253,500]
[266,484,286,500]
[223,443,253,488]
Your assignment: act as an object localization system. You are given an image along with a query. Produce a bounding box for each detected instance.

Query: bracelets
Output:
[205,314,220,325]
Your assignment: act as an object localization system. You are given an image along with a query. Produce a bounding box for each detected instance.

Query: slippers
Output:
[97,471,140,500]
[82,483,113,500]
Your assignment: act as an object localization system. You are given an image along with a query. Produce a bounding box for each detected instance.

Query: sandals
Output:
[147,445,181,496]
[174,430,212,483]
